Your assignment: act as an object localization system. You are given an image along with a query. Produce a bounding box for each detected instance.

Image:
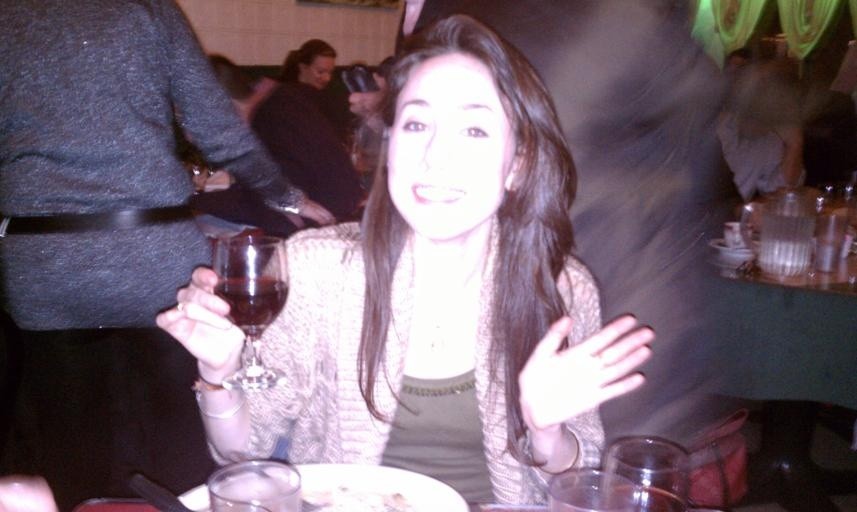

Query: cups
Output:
[546,436,691,512]
[207,460,304,512]
[342,64,377,93]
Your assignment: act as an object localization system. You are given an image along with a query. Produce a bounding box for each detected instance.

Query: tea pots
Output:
[724,184,836,282]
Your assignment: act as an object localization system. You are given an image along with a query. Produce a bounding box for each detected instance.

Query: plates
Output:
[170,463,469,512]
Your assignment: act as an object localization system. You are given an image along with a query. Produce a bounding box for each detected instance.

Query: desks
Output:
[688,241,857,512]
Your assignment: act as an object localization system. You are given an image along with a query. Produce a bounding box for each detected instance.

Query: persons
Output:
[0,0,857,512]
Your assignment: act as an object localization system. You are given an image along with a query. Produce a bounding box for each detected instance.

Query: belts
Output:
[0,206,193,233]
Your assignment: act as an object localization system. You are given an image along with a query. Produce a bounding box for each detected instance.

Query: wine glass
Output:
[212,235,291,393]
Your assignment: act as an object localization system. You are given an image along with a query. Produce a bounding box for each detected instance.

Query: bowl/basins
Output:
[709,240,760,268]
